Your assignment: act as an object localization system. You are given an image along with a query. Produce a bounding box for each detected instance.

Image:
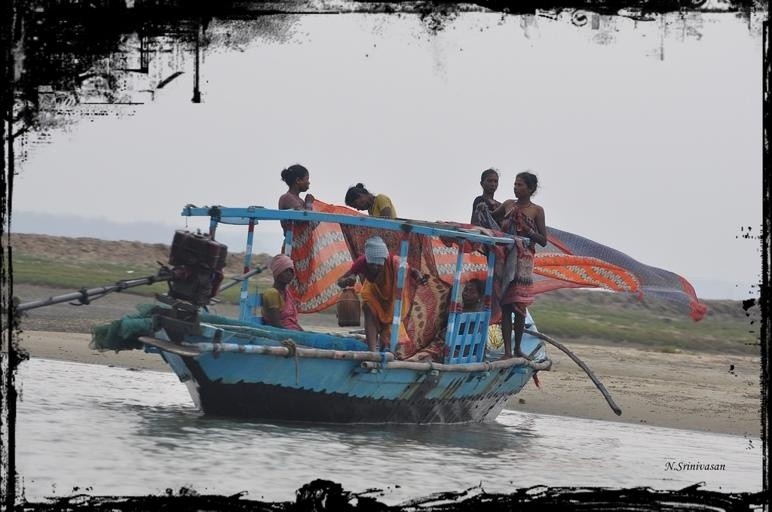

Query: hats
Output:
[270,255,295,282]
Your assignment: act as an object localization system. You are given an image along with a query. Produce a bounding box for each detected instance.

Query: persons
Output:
[472,169,501,229]
[336,236,429,363]
[262,254,304,332]
[474,172,546,363]
[344,184,398,222]
[276,164,322,297]
[408,267,487,366]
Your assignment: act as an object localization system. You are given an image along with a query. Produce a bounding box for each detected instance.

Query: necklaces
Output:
[516,198,533,209]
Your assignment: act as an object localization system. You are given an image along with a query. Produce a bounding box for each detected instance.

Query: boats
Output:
[131,199,553,426]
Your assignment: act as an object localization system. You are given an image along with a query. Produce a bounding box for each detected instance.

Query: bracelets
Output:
[524,227,535,237]
